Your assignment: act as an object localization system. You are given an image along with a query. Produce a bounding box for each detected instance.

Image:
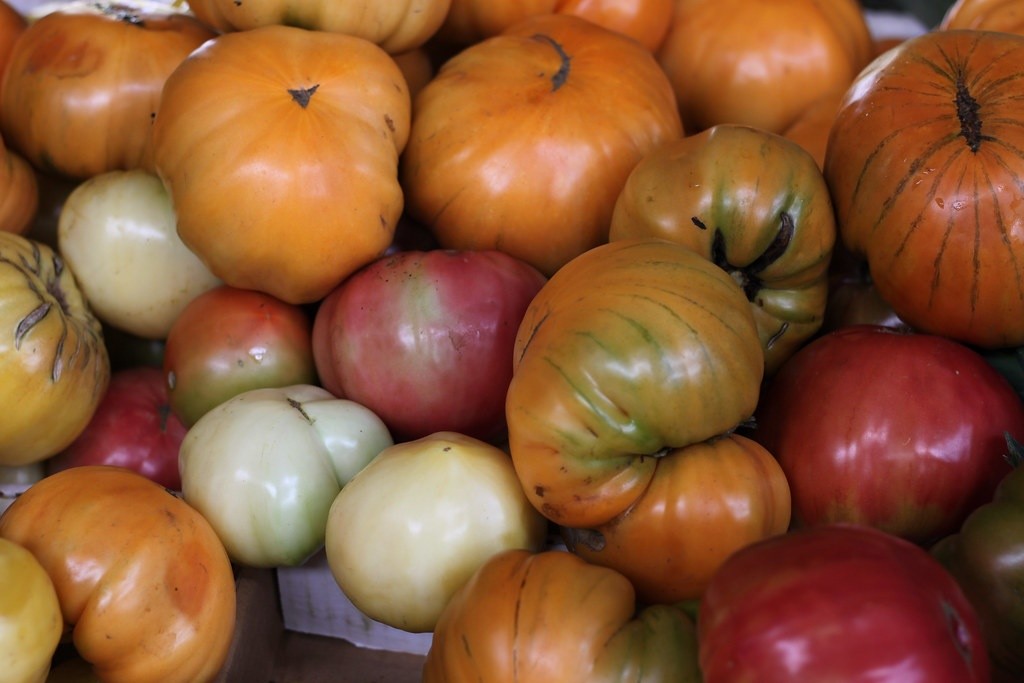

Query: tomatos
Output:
[0,0,1024,683]
